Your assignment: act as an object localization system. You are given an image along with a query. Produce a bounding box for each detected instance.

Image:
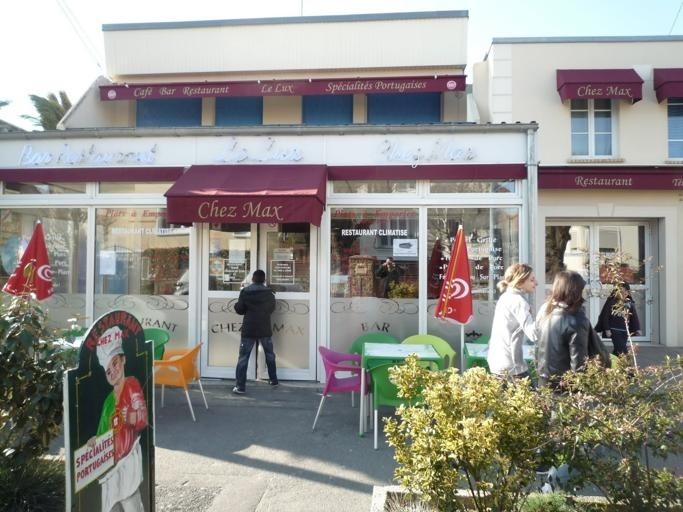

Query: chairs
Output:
[143,328,208,422]
[311,332,457,452]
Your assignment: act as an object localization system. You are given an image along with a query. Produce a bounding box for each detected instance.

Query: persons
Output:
[593,280,643,355]
[535,269,611,398]
[484,261,553,391]
[84,351,148,512]
[374,256,405,298]
[230,269,280,395]
[530,270,569,341]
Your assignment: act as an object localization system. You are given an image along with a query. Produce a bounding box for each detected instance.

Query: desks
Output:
[464,342,535,370]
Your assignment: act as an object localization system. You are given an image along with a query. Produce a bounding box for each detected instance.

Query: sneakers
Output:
[232,386,245,394]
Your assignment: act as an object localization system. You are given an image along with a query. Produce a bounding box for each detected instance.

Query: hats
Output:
[95,325,125,372]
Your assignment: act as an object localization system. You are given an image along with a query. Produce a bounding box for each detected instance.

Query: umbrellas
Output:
[1,219,60,306]
[426,227,451,300]
[430,224,475,376]
